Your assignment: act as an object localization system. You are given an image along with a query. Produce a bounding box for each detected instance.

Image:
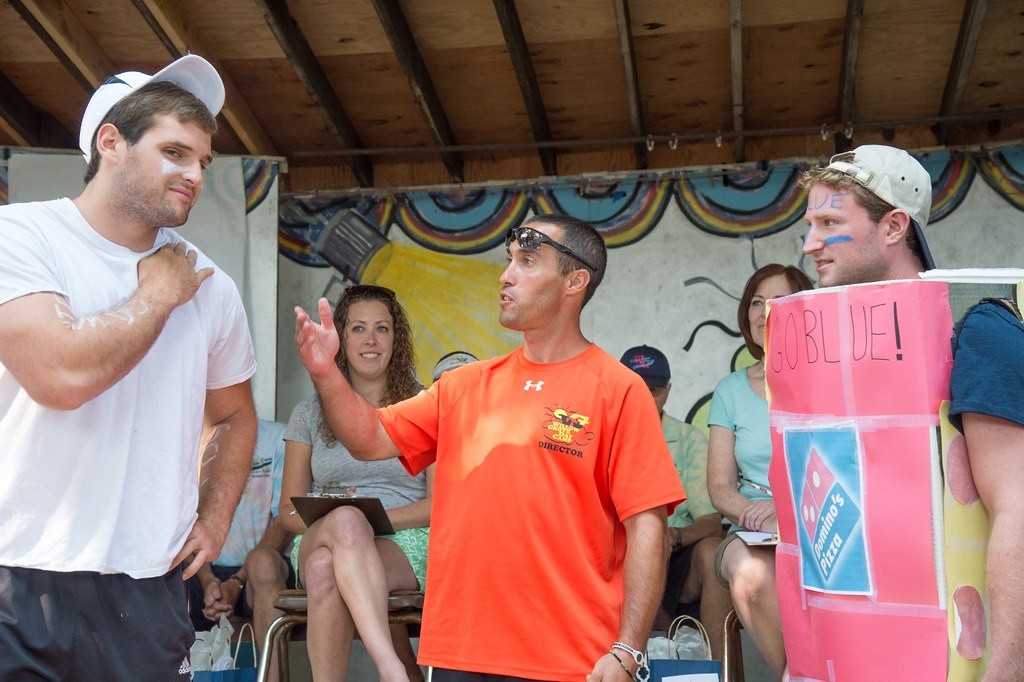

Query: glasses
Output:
[504,226,598,273]
[340,284,395,302]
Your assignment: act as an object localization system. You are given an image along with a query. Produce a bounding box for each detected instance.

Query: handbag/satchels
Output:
[190,622,262,682]
[648,613,724,682]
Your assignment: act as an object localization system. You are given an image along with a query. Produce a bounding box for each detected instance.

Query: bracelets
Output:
[612,642,651,682]
[228,574,247,590]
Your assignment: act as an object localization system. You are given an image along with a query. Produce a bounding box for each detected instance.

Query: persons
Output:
[290,215,688,681]
[279,282,432,682]
[199,413,298,682]
[609,344,737,681]
[702,262,818,678]
[1,48,266,682]
[800,142,1023,681]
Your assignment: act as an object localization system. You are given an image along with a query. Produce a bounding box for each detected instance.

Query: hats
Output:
[826,146,937,270]
[620,344,670,389]
[77,52,225,168]
